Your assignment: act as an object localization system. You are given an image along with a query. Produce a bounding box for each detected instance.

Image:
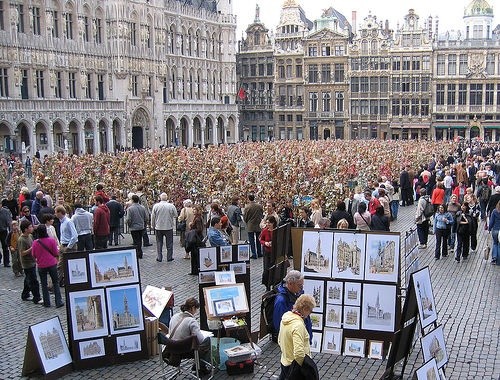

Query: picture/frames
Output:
[321,327,343,355]
[309,332,322,353]
[368,340,384,359]
[343,337,366,357]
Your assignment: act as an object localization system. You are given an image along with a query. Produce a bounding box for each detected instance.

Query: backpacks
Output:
[422,197,434,218]
[261,287,289,333]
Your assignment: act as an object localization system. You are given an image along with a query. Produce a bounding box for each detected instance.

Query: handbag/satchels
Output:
[177,222,186,231]
[484,247,490,260]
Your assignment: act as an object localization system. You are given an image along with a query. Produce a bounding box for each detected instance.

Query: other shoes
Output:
[15,272,23,277]
[418,243,500,266]
[144,243,152,247]
[157,258,162,262]
[4,263,11,268]
[21,295,65,308]
[167,258,174,262]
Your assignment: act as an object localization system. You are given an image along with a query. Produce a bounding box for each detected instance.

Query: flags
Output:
[239,88,246,99]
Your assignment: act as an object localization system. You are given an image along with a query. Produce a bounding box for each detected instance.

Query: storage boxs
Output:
[223,344,255,376]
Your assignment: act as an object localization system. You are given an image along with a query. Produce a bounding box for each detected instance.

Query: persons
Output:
[0,140,500,293]
[127,195,147,259]
[151,192,178,262]
[278,294,317,380]
[273,269,313,345]
[17,220,42,304]
[169,297,211,380]
[93,196,110,250]
[32,224,65,308]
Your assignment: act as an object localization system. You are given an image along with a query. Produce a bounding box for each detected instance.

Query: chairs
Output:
[157,332,214,380]
